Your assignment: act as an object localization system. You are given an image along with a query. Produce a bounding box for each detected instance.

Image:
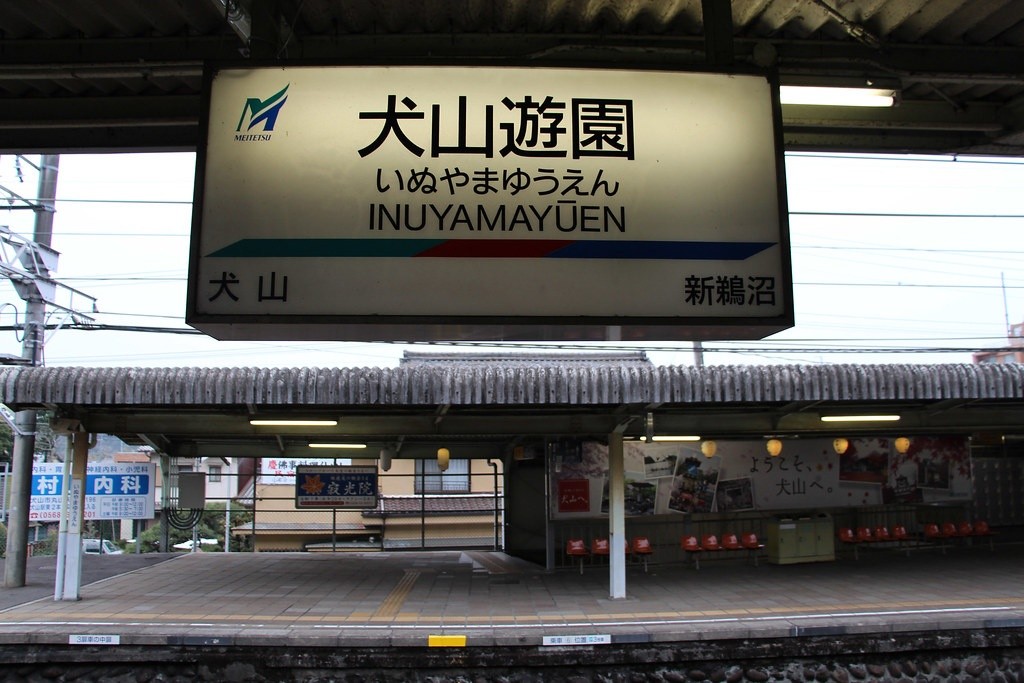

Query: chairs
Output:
[876,527,893,542]
[858,528,875,542]
[624,539,631,555]
[566,539,590,574]
[700,534,722,552]
[942,523,958,536]
[894,526,911,556]
[592,539,610,555]
[743,533,766,566]
[723,534,745,550]
[975,521,995,551]
[924,524,946,553]
[632,537,654,572]
[840,528,863,560]
[957,522,975,537]
[680,535,702,569]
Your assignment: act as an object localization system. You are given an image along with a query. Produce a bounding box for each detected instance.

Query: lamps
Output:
[436,445,450,471]
[380,443,391,471]
[766,438,783,456]
[895,433,910,454]
[833,435,849,453]
[701,441,718,458]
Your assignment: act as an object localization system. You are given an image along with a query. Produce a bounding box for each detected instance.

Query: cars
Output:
[82,538,123,555]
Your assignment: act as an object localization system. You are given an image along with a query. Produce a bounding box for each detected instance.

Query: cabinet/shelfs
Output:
[767,517,835,564]
